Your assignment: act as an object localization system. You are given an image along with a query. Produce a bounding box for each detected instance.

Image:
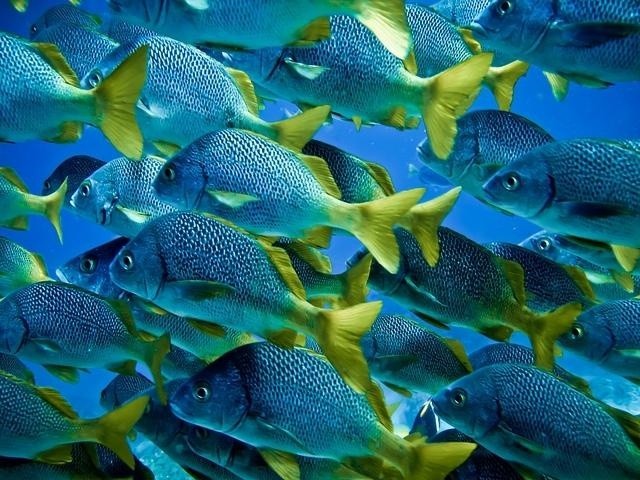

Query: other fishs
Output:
[0,1,640,480]
